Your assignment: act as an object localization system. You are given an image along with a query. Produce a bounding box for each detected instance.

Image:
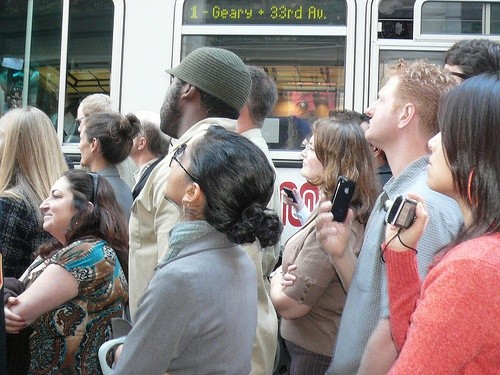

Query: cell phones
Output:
[331,176,355,222]
[283,187,298,203]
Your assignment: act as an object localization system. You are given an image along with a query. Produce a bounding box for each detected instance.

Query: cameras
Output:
[382,194,418,230]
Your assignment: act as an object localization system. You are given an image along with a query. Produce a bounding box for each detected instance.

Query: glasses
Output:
[168,143,200,185]
[74,114,87,126]
[87,172,99,205]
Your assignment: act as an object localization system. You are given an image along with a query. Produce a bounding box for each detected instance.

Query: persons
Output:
[314,57,464,374]
[268,117,380,375]
[359,114,394,194]
[280,108,370,229]
[0,105,67,375]
[379,69,499,374]
[127,107,172,201]
[443,39,500,85]
[75,92,135,188]
[79,109,143,225]
[238,65,283,374]
[111,122,284,375]
[129,47,278,375]
[2,168,131,375]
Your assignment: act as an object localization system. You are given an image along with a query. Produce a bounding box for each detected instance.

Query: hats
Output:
[165,46,252,112]
